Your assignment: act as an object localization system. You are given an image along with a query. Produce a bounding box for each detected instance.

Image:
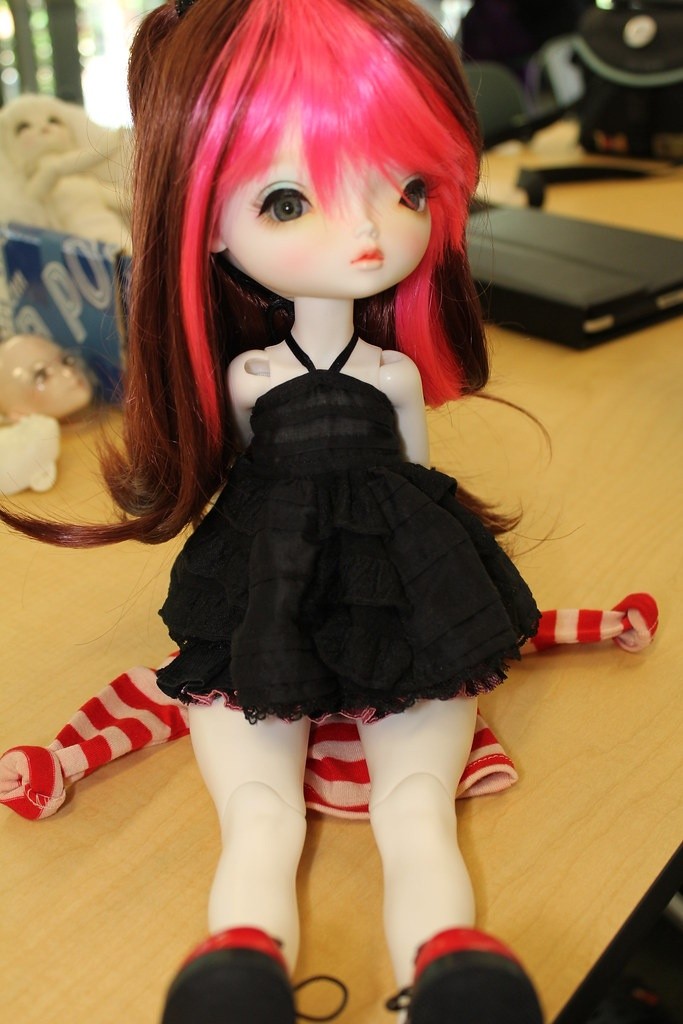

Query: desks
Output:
[0,122,683,1024]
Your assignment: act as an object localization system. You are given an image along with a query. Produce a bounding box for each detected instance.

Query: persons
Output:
[1,1,545,1023]
[1,91,134,260]
[1,334,93,424]
